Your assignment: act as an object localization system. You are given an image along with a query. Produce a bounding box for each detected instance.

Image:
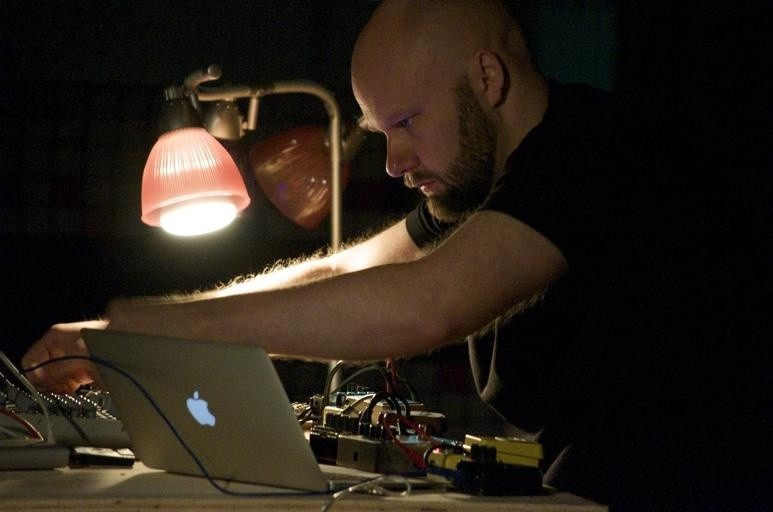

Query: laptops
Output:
[80,327,451,493]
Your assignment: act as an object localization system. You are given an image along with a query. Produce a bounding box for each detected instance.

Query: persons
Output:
[21,1,771,510]
